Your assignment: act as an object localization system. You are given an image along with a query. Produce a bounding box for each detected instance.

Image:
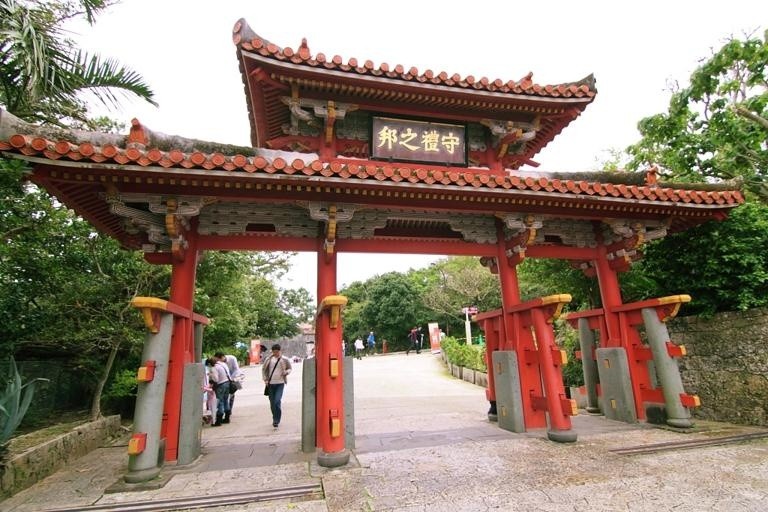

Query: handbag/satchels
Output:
[263,382,270,396]
[229,381,238,394]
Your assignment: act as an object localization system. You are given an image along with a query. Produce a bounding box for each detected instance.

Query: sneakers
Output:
[273,423,278,428]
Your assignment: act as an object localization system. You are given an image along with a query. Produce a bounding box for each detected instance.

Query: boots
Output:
[211,409,231,427]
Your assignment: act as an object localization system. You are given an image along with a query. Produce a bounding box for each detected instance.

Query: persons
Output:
[260,345,267,360]
[342,339,346,358]
[261,344,292,427]
[439,328,445,337]
[366,332,378,357]
[417,323,422,340]
[354,335,365,360]
[204,351,239,426]
[406,326,421,354]
